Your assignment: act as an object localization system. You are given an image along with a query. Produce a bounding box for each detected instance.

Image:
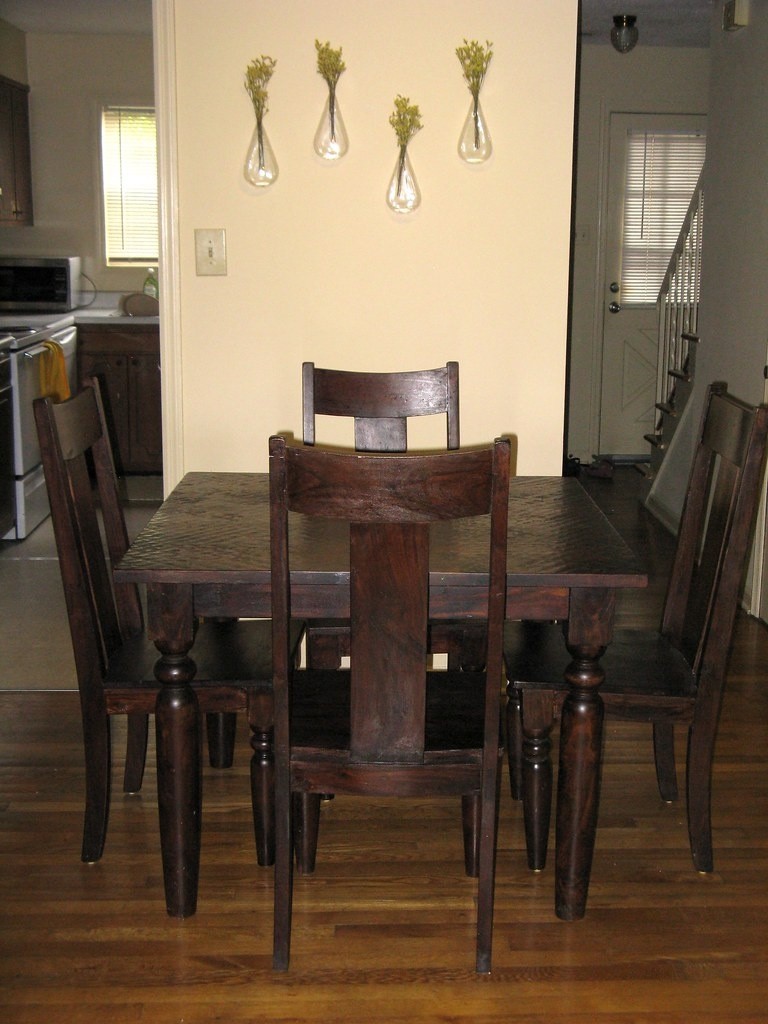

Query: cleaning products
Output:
[142,268,159,299]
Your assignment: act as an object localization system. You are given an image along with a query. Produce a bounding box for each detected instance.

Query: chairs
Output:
[25,361,768,971]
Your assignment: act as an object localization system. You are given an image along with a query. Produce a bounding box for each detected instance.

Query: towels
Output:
[39,341,71,402]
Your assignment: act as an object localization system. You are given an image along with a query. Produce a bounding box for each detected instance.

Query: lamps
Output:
[610,14,639,54]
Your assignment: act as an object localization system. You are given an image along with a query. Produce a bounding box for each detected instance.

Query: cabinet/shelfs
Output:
[76,325,164,473]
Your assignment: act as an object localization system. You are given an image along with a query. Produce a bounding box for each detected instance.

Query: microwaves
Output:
[0,254,81,313]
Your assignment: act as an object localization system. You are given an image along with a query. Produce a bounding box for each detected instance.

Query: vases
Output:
[242,117,280,186]
[385,136,424,214]
[313,90,350,160]
[455,84,494,164]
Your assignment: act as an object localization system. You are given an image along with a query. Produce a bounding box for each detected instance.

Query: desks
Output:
[120,471,656,914]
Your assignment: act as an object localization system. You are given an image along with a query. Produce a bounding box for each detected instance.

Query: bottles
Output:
[143,267,158,299]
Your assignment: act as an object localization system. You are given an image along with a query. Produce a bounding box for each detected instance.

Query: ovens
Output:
[10,327,79,539]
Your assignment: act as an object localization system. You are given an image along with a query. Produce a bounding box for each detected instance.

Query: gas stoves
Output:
[0,315,75,348]
